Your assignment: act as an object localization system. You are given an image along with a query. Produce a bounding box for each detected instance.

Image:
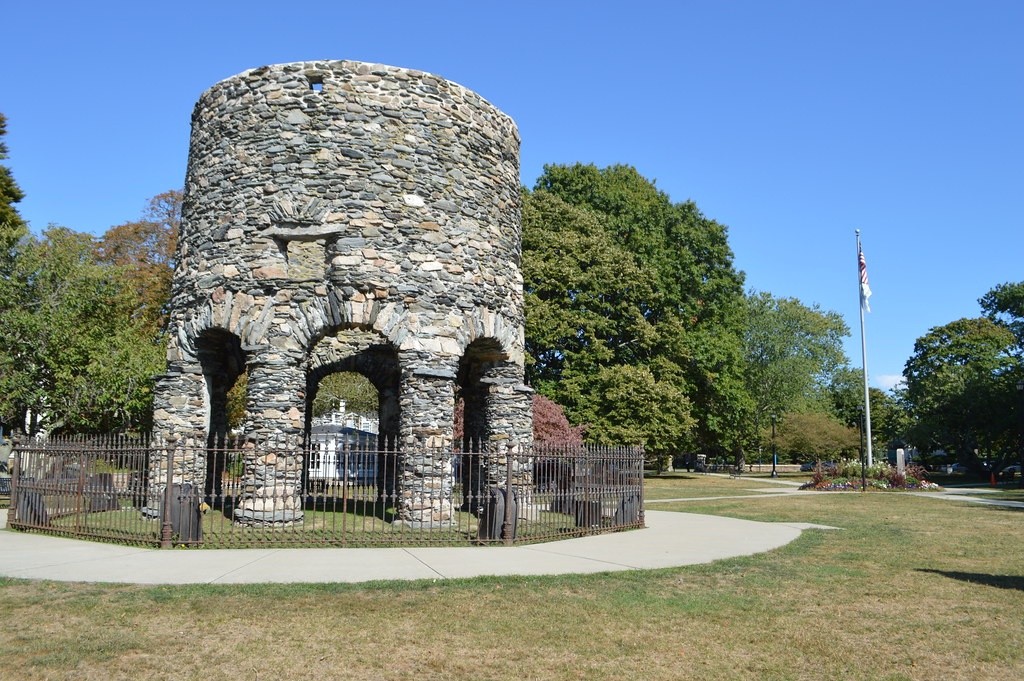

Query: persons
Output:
[685,452,691,472]
[0,438,25,476]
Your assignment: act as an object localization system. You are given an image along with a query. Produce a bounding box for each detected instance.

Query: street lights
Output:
[771,412,778,478]
[857,405,866,493]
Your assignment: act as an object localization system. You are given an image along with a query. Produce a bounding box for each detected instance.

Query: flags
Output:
[858,240,872,312]
